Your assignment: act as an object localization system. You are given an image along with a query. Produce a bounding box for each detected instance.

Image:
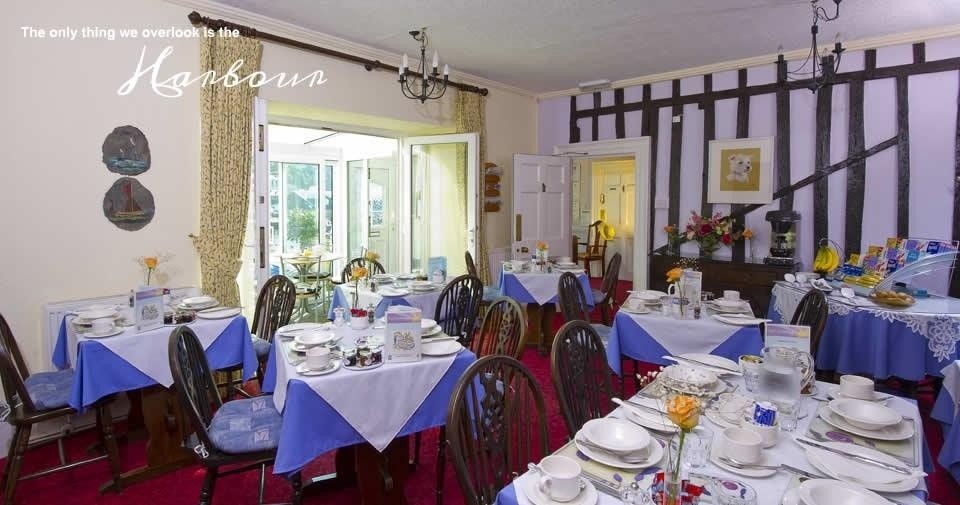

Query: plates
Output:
[369,273,438,297]
[71,296,242,339]
[620,289,765,326]
[277,318,463,376]
[522,352,920,505]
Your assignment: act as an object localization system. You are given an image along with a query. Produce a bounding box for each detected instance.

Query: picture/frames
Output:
[706,136,774,205]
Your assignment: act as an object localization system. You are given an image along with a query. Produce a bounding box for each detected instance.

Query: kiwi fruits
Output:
[870,290,912,304]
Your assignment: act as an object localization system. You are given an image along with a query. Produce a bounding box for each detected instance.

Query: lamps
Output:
[776,0,848,96]
[398,32,451,105]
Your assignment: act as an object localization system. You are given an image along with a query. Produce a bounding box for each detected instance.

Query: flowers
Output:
[685,210,752,251]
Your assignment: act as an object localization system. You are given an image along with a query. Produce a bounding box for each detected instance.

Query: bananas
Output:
[812,245,839,272]
